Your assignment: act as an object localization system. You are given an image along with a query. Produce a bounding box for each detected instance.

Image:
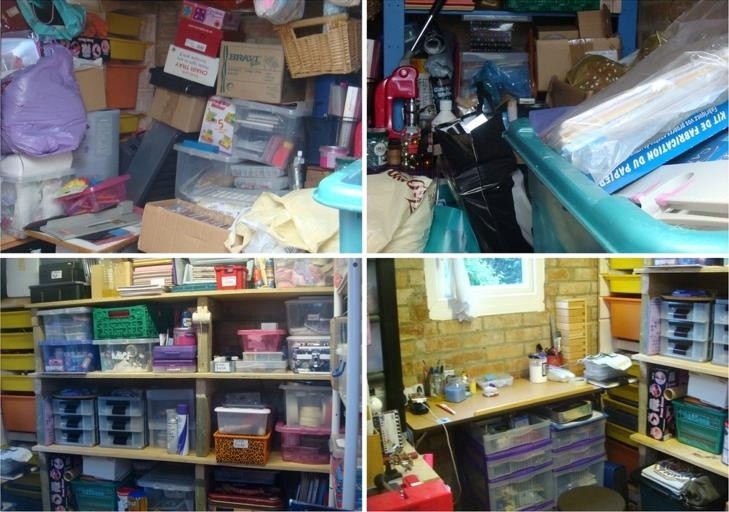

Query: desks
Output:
[404,371,600,477]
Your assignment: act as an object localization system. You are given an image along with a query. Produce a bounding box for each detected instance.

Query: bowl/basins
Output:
[173,327,196,346]
[318,145,350,170]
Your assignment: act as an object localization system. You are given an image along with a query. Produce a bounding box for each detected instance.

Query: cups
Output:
[546,355,563,367]
[287,162,305,191]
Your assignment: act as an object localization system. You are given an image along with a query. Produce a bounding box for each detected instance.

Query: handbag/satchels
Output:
[680,476,721,505]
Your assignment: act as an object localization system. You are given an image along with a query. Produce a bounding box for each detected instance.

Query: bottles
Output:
[80,348,96,369]
[445,376,467,403]
[429,374,445,397]
[180,310,192,327]
[115,487,137,512]
[431,100,456,162]
[335,156,357,173]
[127,490,148,512]
[400,97,422,172]
[387,139,401,165]
[529,352,548,384]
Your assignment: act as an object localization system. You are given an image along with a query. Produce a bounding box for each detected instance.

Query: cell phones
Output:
[409,391,428,404]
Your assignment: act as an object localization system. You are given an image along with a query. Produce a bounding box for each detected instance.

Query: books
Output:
[538,37,729,231]
[118,257,177,299]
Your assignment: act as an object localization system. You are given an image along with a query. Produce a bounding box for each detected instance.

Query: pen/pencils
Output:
[436,404,453,414]
[422,359,443,373]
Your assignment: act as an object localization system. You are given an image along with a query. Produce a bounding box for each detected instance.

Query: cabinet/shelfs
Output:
[24,259,362,511]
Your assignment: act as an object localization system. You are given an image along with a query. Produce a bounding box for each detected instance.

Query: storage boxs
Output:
[597,258,729,511]
[466,410,608,511]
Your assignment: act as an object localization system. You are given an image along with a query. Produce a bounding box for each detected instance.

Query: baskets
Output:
[671,400,726,455]
[213,430,272,465]
[273,12,361,80]
[92,303,174,340]
